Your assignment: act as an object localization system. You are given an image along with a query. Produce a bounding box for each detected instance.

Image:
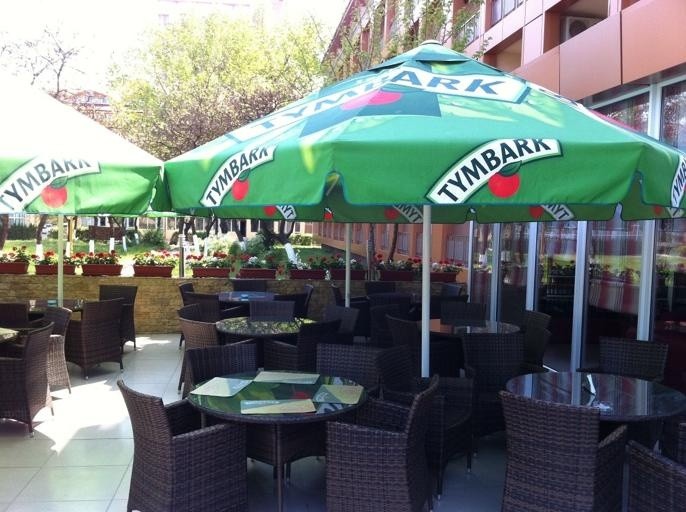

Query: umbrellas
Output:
[167,39,685,378]
[0,93,170,315]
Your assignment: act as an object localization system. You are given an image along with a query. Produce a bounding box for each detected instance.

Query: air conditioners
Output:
[559,15,604,41]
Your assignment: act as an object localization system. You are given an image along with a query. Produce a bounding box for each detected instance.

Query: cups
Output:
[29,300,35,307]
[48,300,56,307]
[77,298,84,307]
[241,294,248,301]
